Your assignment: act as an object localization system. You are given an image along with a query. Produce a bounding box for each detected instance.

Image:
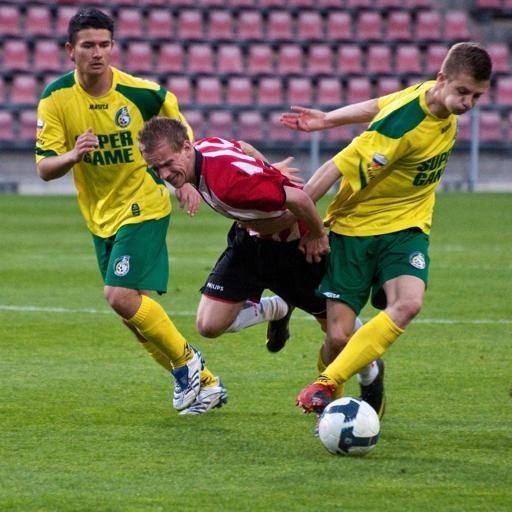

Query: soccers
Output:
[319,395,381,456]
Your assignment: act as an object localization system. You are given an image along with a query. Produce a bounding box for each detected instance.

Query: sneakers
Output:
[265,299,295,353]
[360,357,386,422]
[178,375,228,416]
[171,344,206,411]
[294,381,337,437]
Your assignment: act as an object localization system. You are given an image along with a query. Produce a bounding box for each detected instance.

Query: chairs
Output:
[0,0,510,152]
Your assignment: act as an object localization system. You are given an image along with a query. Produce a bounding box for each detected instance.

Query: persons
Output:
[135,114,388,425]
[34,8,231,414]
[275,41,494,410]
